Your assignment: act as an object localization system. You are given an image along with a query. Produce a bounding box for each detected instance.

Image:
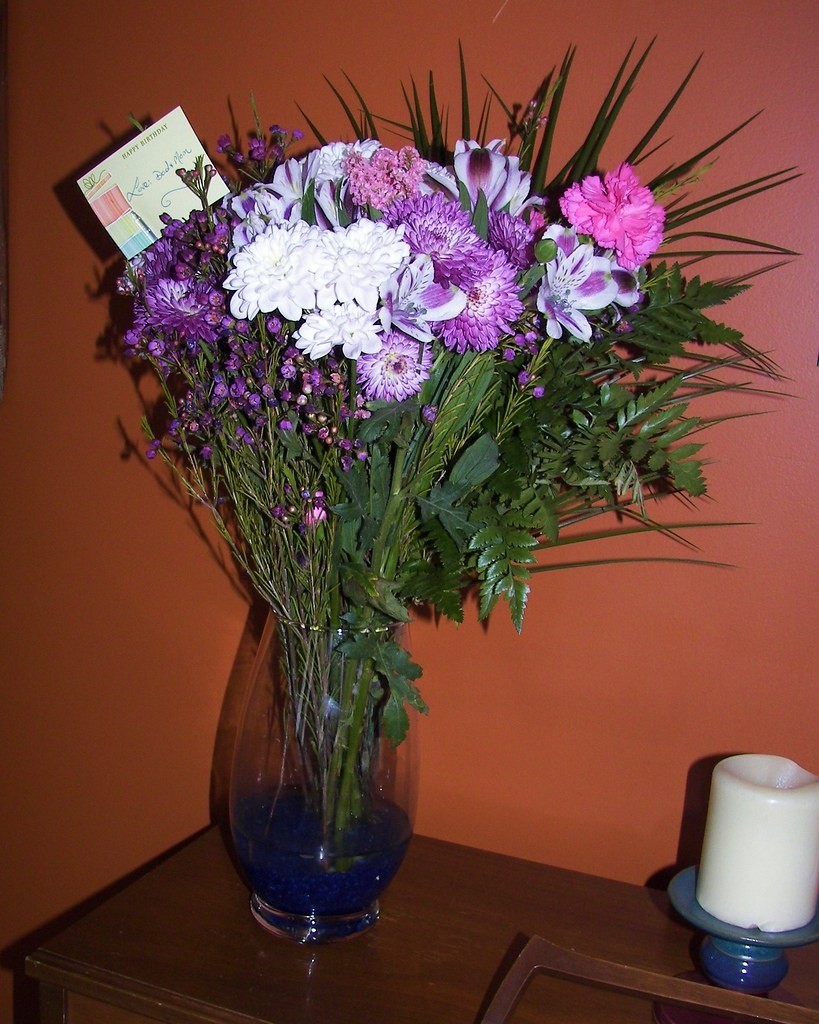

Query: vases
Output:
[207,594,422,942]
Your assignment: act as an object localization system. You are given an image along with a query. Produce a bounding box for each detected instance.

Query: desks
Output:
[21,821,819,1024]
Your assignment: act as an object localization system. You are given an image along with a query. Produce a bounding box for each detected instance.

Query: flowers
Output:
[73,34,810,624]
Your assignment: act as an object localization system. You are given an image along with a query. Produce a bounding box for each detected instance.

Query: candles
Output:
[695,754,819,932]
